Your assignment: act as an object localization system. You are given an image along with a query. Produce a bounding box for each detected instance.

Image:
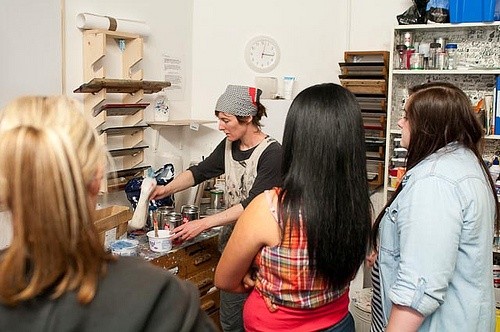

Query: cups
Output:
[210,190,225,209]
[390,169,397,177]
[282,77,294,100]
[390,176,397,187]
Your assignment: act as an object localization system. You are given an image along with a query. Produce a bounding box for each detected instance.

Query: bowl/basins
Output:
[146,230,175,253]
[110,239,139,257]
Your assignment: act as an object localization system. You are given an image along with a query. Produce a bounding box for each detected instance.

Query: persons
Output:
[150,85,283,332]
[367,82,498,332]
[214,83,373,332]
[0,96,214,332]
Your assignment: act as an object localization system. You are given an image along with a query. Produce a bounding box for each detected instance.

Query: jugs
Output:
[256,77,278,99]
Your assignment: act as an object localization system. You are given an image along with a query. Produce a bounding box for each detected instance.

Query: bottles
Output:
[396,30,458,70]
[214,179,226,190]
[128,167,158,229]
[154,100,169,121]
[397,167,406,180]
[203,180,214,198]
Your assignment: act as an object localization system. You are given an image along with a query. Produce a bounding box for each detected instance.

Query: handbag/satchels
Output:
[123,162,175,211]
[395,0,431,25]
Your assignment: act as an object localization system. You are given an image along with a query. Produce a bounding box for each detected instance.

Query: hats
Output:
[215,84,259,117]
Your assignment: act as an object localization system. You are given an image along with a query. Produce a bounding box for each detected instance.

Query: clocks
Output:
[245,36,280,73]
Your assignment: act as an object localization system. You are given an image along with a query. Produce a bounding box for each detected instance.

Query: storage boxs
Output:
[448,0,497,23]
[95,205,133,245]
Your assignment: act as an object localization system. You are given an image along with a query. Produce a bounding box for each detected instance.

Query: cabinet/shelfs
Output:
[136,230,223,332]
[83,29,144,194]
[383,21,500,209]
